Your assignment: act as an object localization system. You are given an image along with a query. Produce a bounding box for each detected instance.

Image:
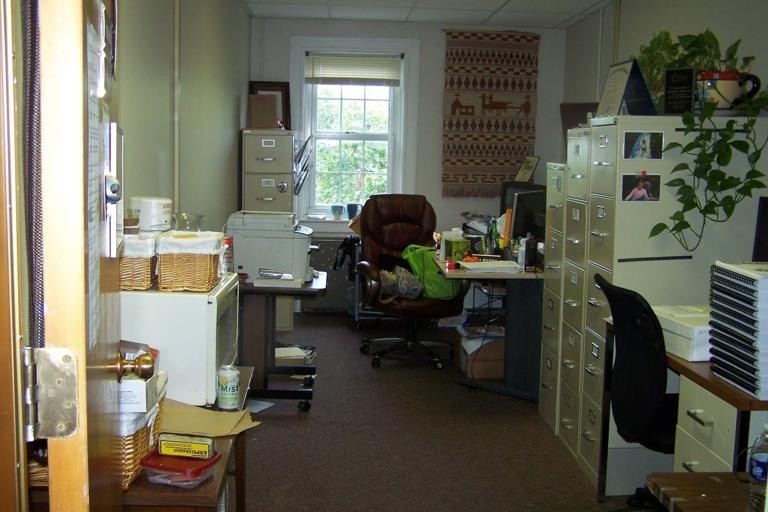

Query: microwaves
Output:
[120,272,242,411]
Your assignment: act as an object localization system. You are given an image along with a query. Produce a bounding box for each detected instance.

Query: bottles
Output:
[747,423,767,510]
[489,218,499,253]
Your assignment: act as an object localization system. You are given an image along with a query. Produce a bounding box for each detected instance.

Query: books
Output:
[457,260,520,272]
[708,258,767,401]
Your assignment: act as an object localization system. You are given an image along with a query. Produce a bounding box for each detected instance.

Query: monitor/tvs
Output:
[499,181,546,219]
[509,189,546,243]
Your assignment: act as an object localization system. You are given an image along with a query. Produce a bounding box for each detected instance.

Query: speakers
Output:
[524,238,538,272]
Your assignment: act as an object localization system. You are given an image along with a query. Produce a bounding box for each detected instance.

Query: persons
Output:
[625,180,651,201]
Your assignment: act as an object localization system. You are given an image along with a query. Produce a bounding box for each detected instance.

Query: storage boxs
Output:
[459,326,506,379]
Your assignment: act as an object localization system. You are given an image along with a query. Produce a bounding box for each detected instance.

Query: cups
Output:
[346,203,362,220]
[123,207,140,235]
[331,205,345,221]
[172,211,204,231]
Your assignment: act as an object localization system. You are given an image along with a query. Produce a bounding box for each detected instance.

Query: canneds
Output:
[215,364,242,411]
[221,235,235,273]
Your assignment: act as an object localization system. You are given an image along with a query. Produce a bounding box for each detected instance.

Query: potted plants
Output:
[646,28,768,257]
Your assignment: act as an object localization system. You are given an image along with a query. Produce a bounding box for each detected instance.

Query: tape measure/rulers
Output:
[445,260,460,269]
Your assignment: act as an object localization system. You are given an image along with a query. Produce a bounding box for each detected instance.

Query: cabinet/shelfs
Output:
[239,128,302,214]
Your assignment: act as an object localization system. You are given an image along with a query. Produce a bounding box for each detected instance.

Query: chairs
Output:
[351,190,454,373]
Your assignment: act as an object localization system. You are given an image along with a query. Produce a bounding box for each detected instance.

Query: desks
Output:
[115,362,255,511]
[430,254,545,401]
[235,271,330,412]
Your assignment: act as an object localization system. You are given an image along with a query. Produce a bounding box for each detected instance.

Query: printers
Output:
[225,211,320,283]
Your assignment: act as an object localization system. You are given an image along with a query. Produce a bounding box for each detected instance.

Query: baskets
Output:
[119,231,158,291]
[155,230,224,292]
[28,372,169,492]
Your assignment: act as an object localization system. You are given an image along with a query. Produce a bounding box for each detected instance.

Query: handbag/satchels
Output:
[333,235,462,301]
